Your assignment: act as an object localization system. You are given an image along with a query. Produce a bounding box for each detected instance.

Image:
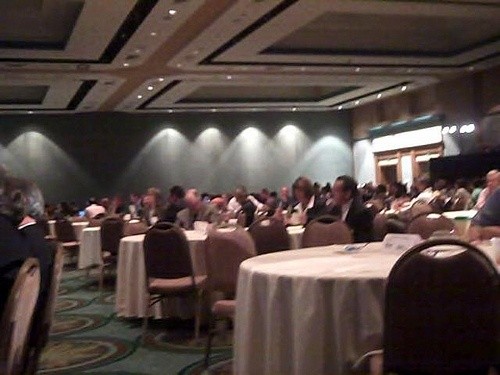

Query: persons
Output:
[200,167,500,244]
[0,164,28,333]
[45,188,161,232]
[158,187,187,223]
[7,178,47,254]
[174,188,224,230]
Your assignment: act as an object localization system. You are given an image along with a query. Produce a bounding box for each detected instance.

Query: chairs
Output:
[1,182,500,375]
